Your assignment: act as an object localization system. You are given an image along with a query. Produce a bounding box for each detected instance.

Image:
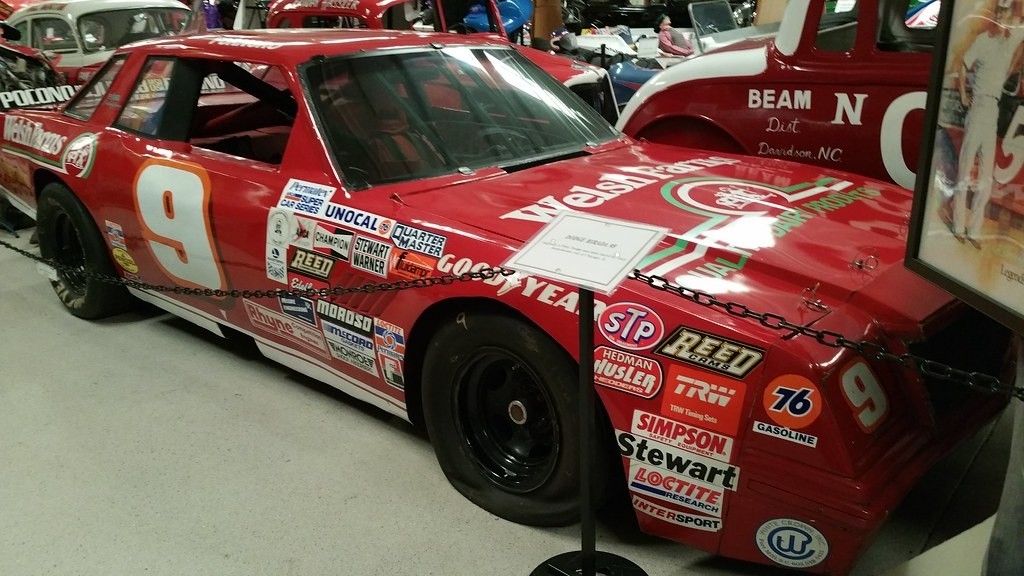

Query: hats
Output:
[996,0,1013,9]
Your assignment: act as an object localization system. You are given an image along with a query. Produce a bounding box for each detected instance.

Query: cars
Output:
[0,0,1020,575]
[0,0,939,124]
[616,0,952,192]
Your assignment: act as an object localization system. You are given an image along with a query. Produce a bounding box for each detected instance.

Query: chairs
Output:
[189,94,446,176]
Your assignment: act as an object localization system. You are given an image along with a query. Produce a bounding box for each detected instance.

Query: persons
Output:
[653,13,694,55]
[951,0,1024,245]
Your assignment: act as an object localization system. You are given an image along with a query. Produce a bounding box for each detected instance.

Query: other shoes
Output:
[949,224,982,251]
